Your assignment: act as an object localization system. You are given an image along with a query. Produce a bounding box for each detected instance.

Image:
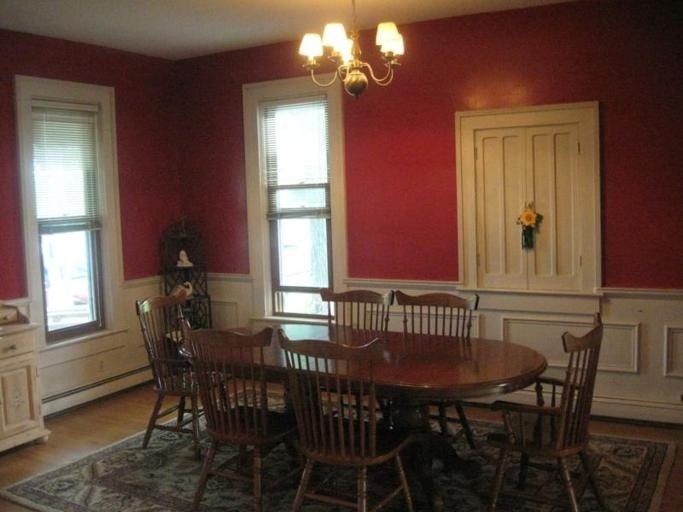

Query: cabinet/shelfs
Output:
[0,323,52,452]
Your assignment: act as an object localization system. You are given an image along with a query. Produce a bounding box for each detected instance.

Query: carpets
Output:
[0,386,678,510]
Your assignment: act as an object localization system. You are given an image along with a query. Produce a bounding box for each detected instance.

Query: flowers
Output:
[516,206,542,248]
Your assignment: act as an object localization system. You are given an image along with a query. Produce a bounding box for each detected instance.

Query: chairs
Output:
[487,312,605,512]
[181,326,306,511]
[134,285,230,461]
[320,290,480,339]
[276,328,413,512]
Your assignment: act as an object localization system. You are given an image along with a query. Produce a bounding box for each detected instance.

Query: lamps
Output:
[298,0,406,103]
